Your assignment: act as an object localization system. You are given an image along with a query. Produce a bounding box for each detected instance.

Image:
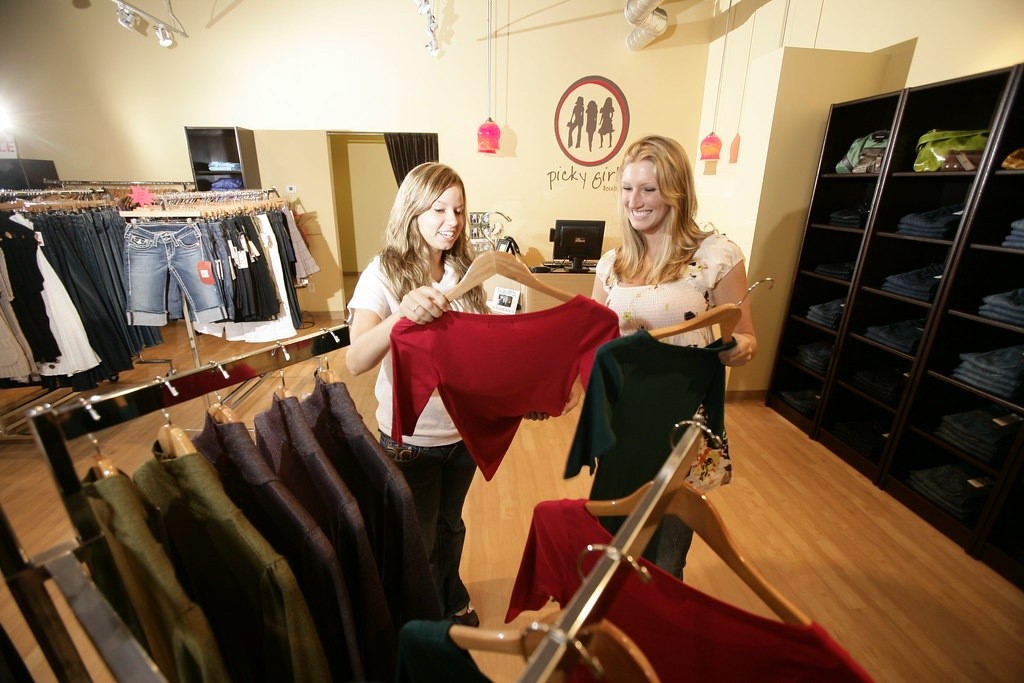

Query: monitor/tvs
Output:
[549,219,605,272]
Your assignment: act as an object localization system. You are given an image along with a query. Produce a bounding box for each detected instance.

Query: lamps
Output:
[112,0,190,48]
[476,0,501,155]
[700,0,733,161]
[414,0,440,56]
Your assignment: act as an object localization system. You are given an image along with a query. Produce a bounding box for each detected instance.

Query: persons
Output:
[344,160,549,627]
[590,136,757,579]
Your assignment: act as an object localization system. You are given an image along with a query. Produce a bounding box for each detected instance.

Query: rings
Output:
[413,305,420,311]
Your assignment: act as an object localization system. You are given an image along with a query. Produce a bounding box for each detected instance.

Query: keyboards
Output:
[542,260,597,268]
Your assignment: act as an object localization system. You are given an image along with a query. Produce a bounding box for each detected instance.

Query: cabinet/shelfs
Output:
[764,63,1024,599]
[183,126,262,192]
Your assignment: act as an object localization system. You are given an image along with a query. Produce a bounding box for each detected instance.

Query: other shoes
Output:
[452,602,479,627]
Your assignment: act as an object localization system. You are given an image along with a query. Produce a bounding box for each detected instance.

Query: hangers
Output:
[0,180,876,683]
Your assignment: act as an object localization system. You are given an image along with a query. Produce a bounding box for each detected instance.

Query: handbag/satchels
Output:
[671,421,731,495]
[913,128,990,172]
[835,130,890,173]
